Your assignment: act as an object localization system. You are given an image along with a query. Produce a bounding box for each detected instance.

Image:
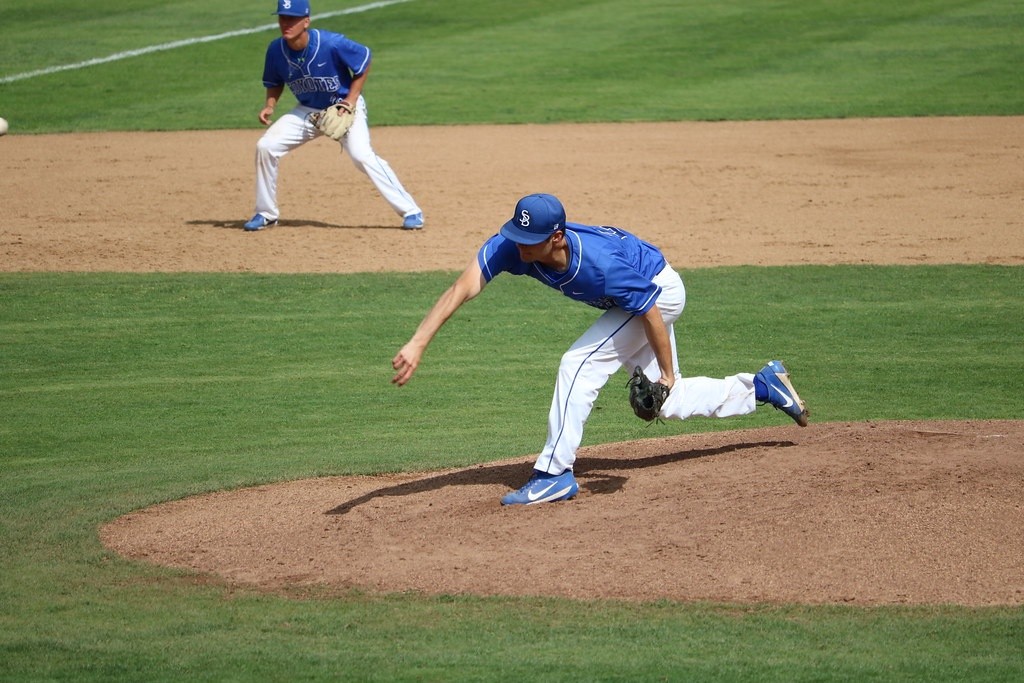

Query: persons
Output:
[392,193,808,506]
[243,0,424,231]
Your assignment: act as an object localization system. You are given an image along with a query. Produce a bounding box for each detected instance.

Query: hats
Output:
[500,193,566,245]
[271,0,310,17]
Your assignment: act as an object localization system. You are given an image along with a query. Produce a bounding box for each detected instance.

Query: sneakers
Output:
[500,469,579,505]
[403,212,424,228]
[755,361,810,427]
[244,214,278,231]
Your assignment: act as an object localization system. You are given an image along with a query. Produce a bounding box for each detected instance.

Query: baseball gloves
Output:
[308,102,356,141]
[629,365,669,421]
